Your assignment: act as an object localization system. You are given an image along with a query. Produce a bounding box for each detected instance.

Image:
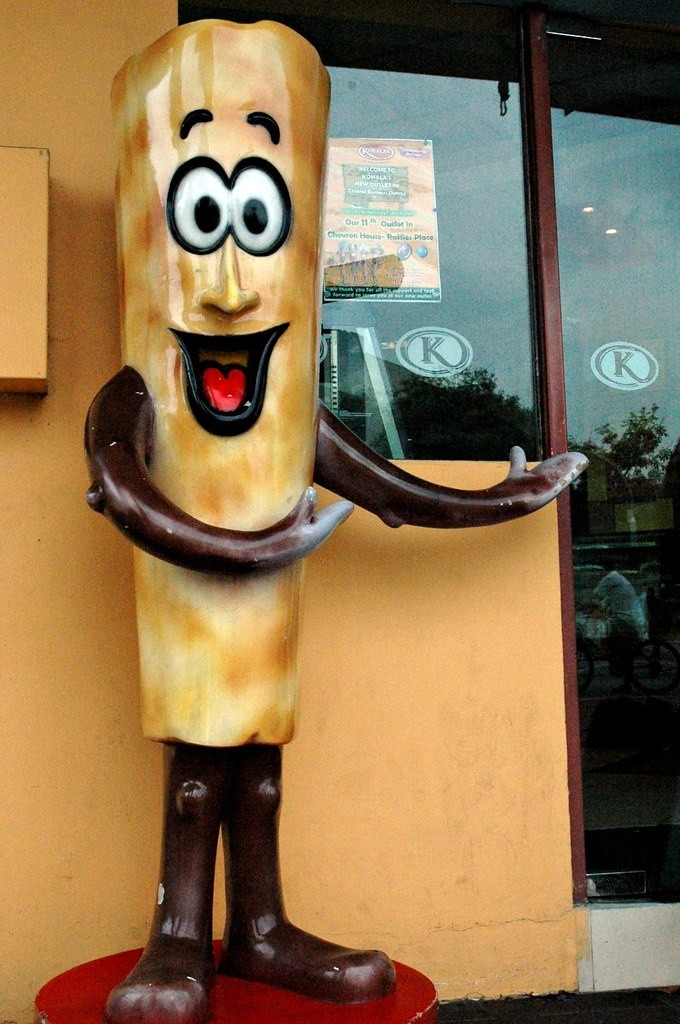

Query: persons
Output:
[574,557,664,695]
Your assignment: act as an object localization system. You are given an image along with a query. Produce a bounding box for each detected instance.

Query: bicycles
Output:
[575,601,680,696]
